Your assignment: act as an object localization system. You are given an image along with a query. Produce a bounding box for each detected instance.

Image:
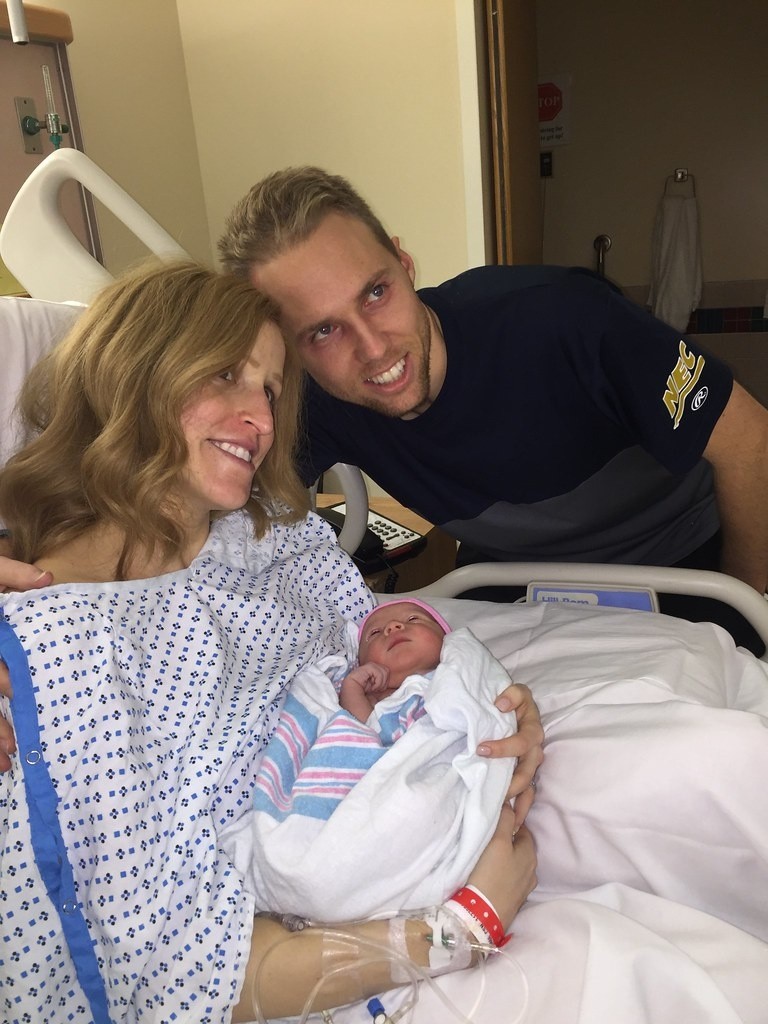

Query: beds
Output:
[0,148,768,1024]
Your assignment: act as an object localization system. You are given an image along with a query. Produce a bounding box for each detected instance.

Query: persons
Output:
[1,169,768,1023]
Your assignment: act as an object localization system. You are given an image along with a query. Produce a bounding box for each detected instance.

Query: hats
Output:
[358,598,452,643]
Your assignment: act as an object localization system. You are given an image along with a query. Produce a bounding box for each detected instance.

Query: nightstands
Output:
[316,492,457,594]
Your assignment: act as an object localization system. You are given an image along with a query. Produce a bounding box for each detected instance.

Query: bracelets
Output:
[451,887,514,946]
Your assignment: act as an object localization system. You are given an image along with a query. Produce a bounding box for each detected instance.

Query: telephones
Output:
[313,501,428,576]
